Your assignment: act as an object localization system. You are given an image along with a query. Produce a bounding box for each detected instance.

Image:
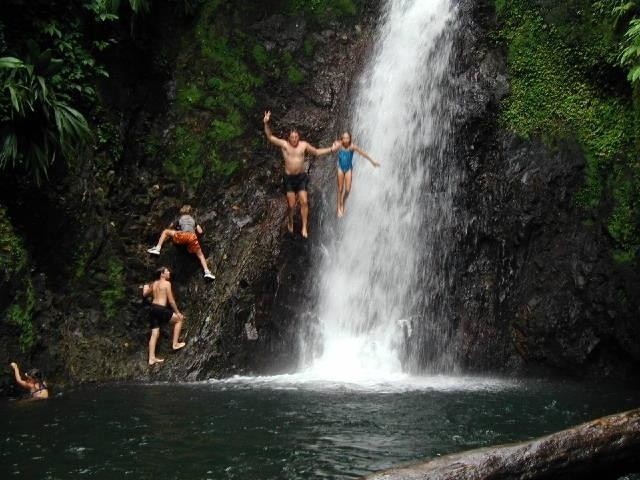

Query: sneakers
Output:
[147,245,161,255]
[204,271,216,280]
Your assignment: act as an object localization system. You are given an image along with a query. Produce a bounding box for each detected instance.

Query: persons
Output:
[261,109,338,242]
[10,361,51,403]
[141,264,187,367]
[331,131,383,218]
[145,204,217,283]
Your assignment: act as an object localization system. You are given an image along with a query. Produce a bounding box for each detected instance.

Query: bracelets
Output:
[330,147,334,153]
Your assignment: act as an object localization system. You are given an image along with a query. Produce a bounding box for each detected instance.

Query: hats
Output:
[25,368,41,379]
